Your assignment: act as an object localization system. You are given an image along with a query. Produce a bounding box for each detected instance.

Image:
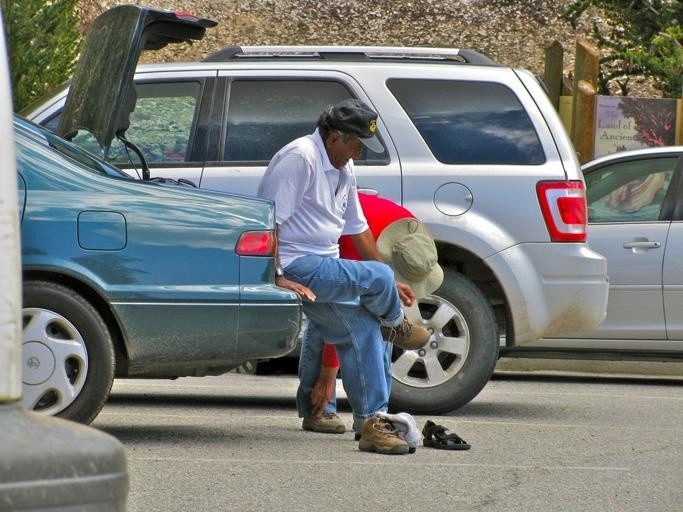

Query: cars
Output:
[12,2,318,425]
[498,140,682,362]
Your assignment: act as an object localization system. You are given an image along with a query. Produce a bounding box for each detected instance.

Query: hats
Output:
[375,217,445,300]
[325,98,387,155]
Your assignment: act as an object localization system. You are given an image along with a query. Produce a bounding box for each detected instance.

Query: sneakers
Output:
[352,416,416,454]
[381,318,431,350]
[302,410,347,434]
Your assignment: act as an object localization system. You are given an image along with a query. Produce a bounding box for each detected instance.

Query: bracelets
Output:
[275,269,284,276]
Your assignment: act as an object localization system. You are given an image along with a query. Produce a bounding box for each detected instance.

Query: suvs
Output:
[18,43,610,420]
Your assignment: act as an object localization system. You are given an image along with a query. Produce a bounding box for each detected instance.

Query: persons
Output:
[255,99,431,455]
[295,192,444,434]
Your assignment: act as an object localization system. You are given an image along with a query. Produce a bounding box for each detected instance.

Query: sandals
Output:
[421,419,470,449]
